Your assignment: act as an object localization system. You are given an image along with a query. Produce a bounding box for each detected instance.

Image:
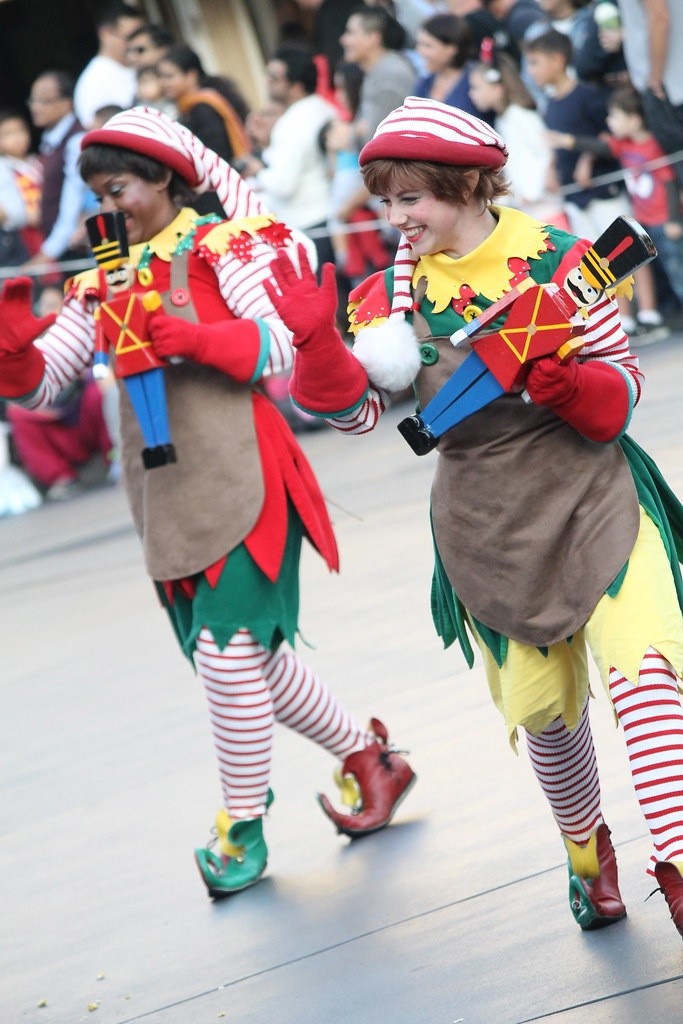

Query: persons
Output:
[1,107,417,903]
[267,95,683,938]
[1,0,683,535]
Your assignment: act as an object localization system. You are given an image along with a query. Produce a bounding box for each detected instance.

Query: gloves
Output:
[263,243,369,418]
[525,357,634,447]
[0,276,57,404]
[147,315,271,386]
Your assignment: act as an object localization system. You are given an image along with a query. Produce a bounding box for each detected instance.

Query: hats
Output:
[358,96,510,177]
[81,106,270,221]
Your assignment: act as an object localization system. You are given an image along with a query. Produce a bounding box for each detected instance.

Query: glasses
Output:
[25,97,63,105]
[125,45,156,54]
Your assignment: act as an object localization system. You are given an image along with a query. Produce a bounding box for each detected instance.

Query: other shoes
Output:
[194,787,275,896]
[625,321,671,349]
[560,824,628,931]
[317,718,418,838]
[644,860,683,940]
